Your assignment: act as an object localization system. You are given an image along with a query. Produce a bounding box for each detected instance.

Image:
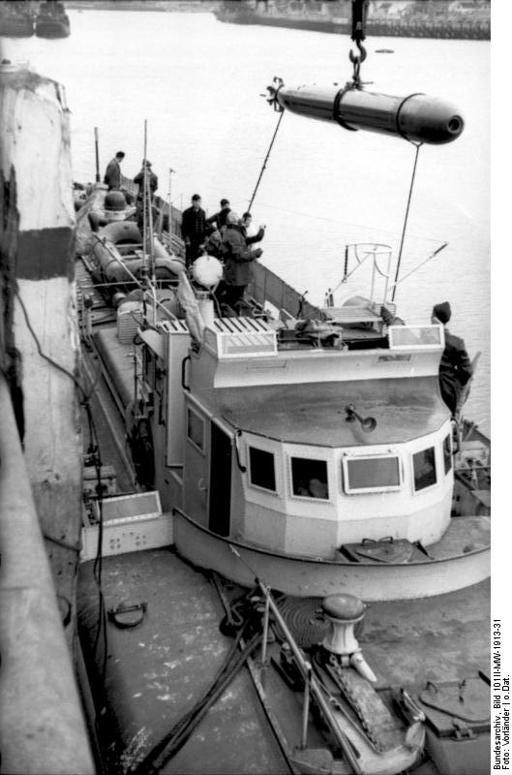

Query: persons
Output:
[179,193,268,304]
[132,160,159,205]
[428,301,470,421]
[105,149,125,189]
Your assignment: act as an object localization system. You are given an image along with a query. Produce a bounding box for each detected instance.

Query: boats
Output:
[73,173,491,775]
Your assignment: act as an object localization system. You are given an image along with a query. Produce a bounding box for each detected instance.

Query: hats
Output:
[434,302,451,325]
